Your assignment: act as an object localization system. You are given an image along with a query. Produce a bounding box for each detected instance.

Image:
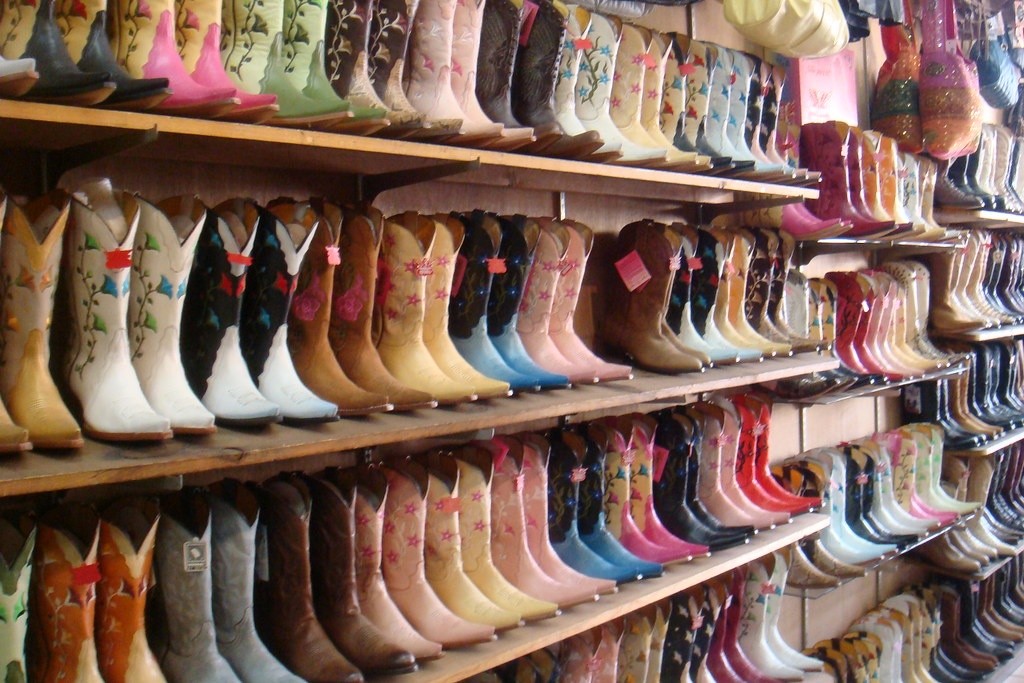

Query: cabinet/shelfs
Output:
[2,96,1023,682]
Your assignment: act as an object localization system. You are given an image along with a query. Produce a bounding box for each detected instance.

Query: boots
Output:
[0,0,1022,683]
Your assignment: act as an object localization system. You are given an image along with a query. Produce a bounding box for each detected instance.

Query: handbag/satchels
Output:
[920,1,983,161]
[875,0,924,154]
[724,0,851,59]
[972,0,1019,111]
[1006,82,1024,136]
